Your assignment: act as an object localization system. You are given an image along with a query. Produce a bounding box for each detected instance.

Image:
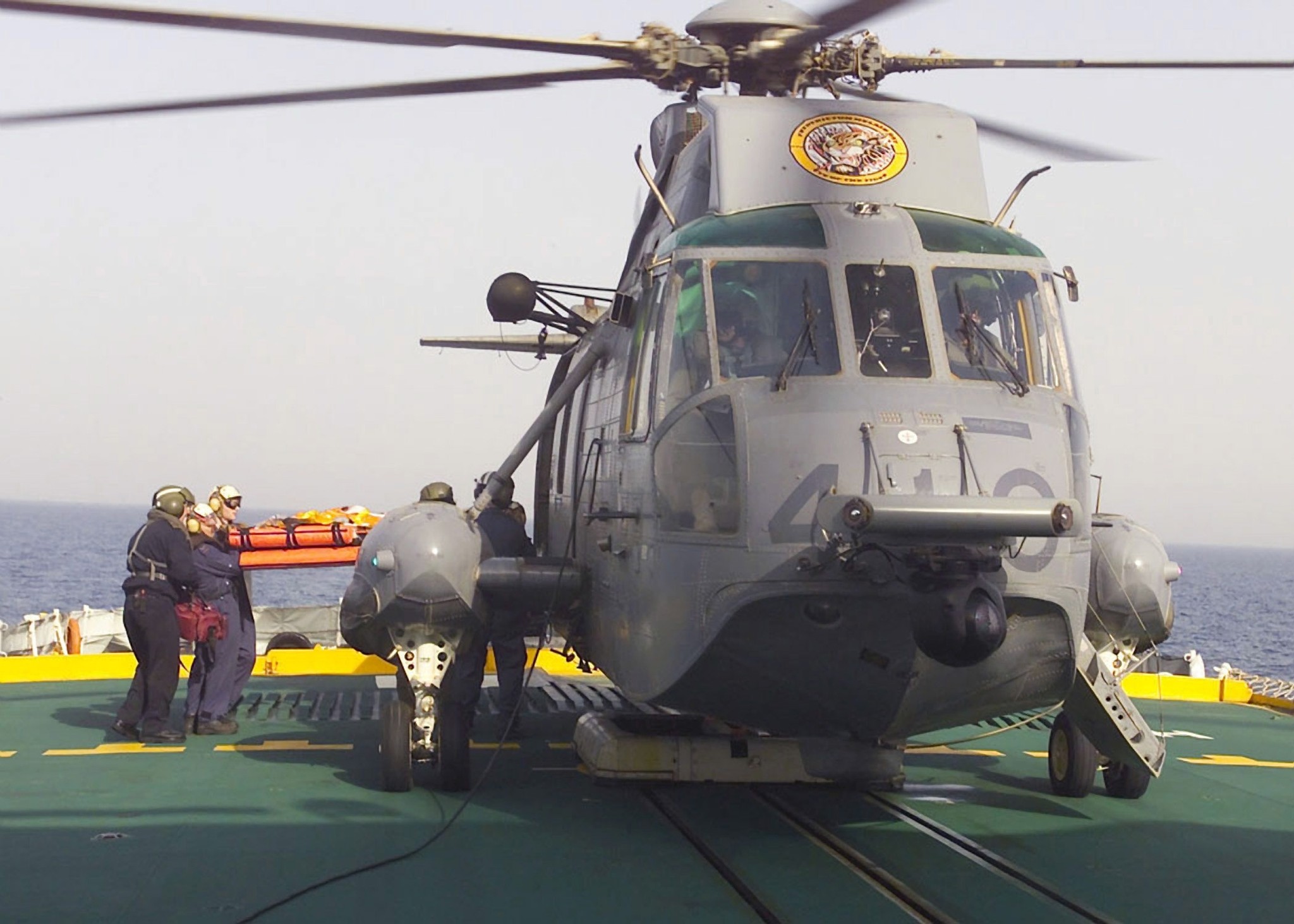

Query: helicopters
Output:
[0,3,1293,800]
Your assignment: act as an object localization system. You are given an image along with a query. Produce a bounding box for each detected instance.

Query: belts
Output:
[136,571,170,582]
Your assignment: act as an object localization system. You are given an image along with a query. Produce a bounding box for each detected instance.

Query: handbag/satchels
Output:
[175,586,228,643]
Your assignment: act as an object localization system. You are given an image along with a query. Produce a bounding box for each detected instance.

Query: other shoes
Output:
[196,715,238,735]
[183,714,196,733]
[493,715,523,738]
[441,764,471,790]
[139,718,186,744]
[112,719,139,740]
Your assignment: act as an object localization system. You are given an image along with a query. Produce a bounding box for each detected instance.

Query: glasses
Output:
[225,499,241,509]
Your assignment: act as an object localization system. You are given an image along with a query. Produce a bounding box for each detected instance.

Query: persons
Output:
[183,501,239,734]
[472,471,538,740]
[111,485,202,741]
[207,484,259,723]
[943,291,1018,373]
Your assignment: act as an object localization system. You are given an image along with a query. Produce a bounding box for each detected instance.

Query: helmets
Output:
[716,281,761,324]
[420,482,453,503]
[152,486,195,516]
[207,485,242,512]
[479,471,510,509]
[185,504,213,533]
[949,274,1000,325]
